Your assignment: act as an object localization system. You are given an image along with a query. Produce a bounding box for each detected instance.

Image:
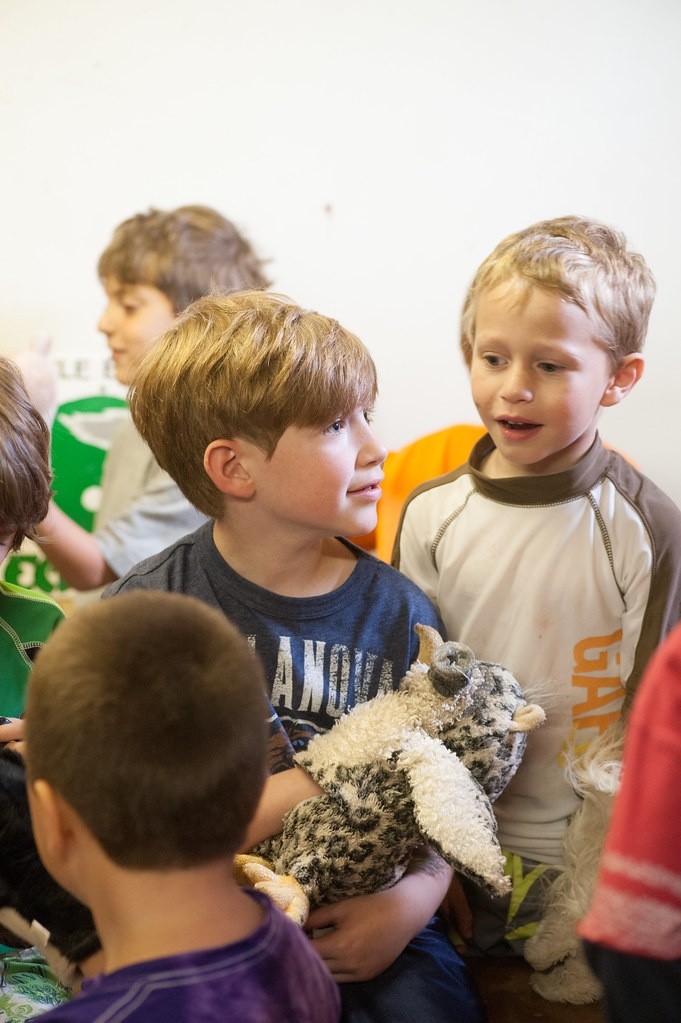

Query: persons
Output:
[0,204,681,1023]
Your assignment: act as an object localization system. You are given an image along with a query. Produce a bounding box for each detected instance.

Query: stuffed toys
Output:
[232,623,547,929]
